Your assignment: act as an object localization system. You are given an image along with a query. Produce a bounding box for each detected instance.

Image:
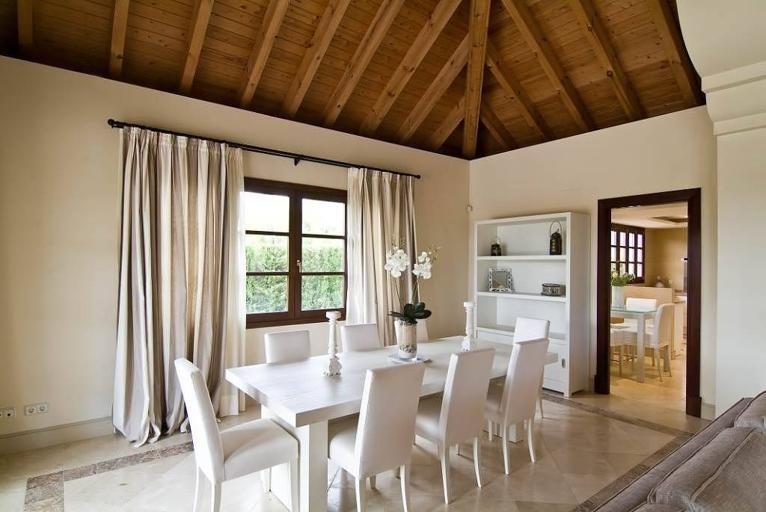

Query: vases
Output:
[393,321,417,360]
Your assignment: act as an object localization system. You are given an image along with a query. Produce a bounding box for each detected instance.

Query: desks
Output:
[609,310,658,383]
[224,335,558,512]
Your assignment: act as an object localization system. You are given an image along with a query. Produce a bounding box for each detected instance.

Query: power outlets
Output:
[24,405,48,415]
[0,409,17,419]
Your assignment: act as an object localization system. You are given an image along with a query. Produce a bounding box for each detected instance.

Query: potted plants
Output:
[611,267,635,309]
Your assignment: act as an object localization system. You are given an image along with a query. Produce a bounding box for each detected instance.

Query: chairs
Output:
[642,303,676,382]
[173,357,300,512]
[625,298,657,370]
[610,323,632,374]
[396,348,496,505]
[341,322,379,352]
[328,362,426,512]
[455,338,550,476]
[514,316,550,419]
[264,330,311,363]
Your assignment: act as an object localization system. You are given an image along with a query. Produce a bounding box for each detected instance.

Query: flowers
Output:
[384,233,443,324]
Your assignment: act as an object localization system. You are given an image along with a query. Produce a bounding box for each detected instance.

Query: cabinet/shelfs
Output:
[472,211,591,398]
